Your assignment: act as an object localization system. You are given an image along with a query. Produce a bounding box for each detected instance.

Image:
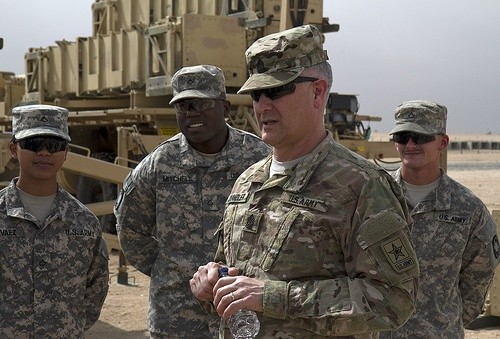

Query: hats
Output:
[12,105,71,142]
[236,24,329,94]
[168,65,225,104]
[389,101,447,135]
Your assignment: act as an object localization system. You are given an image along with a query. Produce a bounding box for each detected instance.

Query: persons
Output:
[113,65,275,339]
[0,105,110,339]
[189,25,421,339]
[379,99,500,339]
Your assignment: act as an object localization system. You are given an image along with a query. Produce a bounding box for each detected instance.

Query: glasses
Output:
[174,99,217,113]
[250,75,320,102]
[15,136,68,153]
[393,132,443,145]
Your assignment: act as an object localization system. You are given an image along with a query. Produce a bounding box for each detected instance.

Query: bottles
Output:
[217,267,261,339]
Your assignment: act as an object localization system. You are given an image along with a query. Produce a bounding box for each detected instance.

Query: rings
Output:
[230,292,235,300]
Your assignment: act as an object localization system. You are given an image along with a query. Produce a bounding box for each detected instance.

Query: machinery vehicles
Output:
[0,0,401,261]
